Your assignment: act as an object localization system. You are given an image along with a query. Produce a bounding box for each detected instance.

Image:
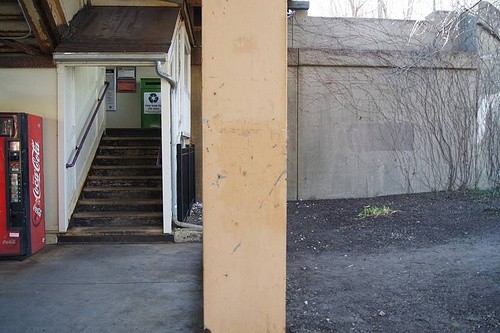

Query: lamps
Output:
[287,0,310,21]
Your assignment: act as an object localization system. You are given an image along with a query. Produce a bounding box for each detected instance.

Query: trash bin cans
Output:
[139,76,161,127]
